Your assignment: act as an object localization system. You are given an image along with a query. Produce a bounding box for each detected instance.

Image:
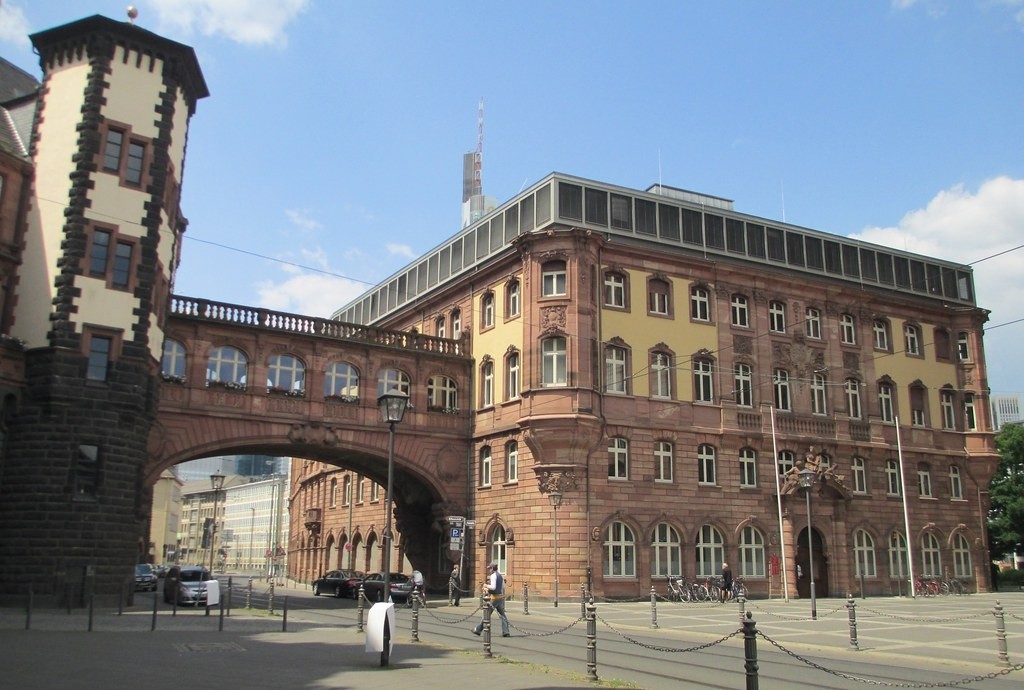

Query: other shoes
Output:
[503,632,510,637]
[454,601,459,606]
[470,629,480,636]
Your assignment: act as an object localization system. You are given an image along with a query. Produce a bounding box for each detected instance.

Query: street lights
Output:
[265,460,276,582]
[375,387,410,666]
[209,470,227,574]
[547,490,563,607]
[797,466,818,620]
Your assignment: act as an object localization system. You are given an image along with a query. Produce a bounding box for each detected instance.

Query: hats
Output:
[486,562,498,569]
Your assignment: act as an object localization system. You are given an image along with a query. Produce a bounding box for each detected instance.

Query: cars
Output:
[152,564,170,578]
[134,563,157,591]
[311,569,365,598]
[352,571,412,603]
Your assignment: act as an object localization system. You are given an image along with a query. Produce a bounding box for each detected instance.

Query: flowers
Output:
[160,369,188,386]
[427,405,460,415]
[326,393,360,404]
[389,400,414,410]
[268,386,306,396]
[207,379,245,392]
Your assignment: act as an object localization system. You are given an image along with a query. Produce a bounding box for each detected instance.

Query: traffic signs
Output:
[445,515,465,522]
[466,520,477,525]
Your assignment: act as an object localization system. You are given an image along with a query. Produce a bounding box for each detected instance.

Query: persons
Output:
[720,562,733,603]
[825,463,851,490]
[993,569,1001,591]
[780,461,802,496]
[805,446,819,471]
[470,562,510,638]
[452,564,461,606]
[408,570,424,596]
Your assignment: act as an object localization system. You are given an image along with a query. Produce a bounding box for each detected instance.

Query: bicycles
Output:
[666,574,750,603]
[908,574,953,597]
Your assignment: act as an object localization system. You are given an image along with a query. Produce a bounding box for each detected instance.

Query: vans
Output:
[164,565,213,606]
